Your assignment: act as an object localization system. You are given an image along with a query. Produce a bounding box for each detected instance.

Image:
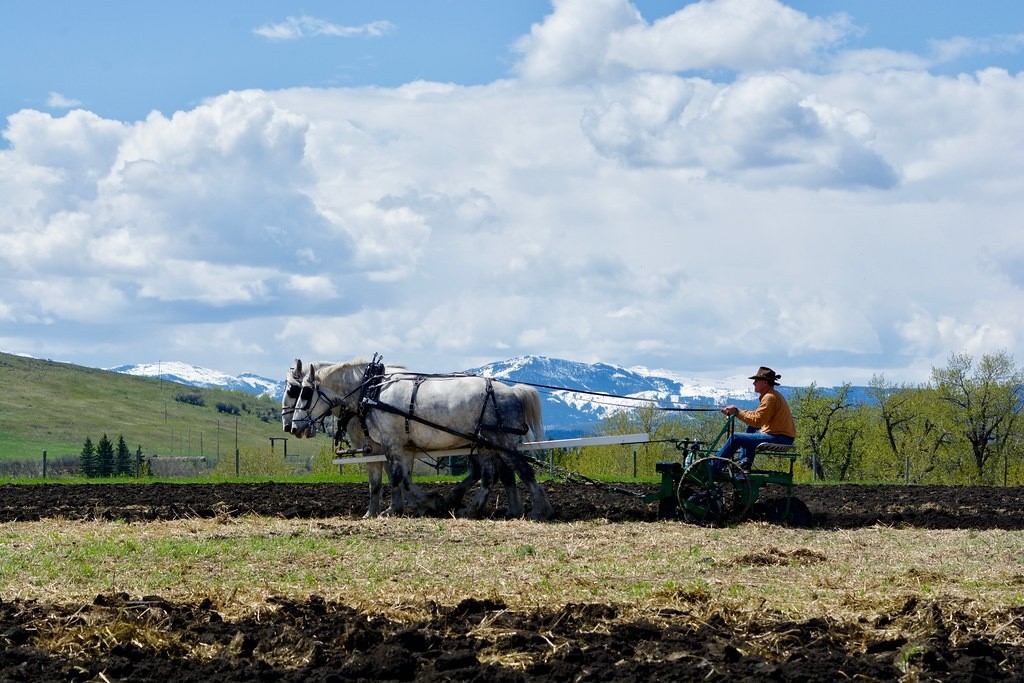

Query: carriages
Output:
[282,352,805,525]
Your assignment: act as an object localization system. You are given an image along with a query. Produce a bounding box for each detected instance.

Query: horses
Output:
[280,357,555,521]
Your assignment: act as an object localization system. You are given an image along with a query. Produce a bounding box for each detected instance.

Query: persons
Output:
[706,366,796,474]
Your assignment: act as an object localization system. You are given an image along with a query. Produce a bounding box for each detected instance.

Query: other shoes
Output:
[731,462,752,470]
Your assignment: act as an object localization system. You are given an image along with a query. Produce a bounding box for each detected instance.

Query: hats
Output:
[748,367,781,386]
